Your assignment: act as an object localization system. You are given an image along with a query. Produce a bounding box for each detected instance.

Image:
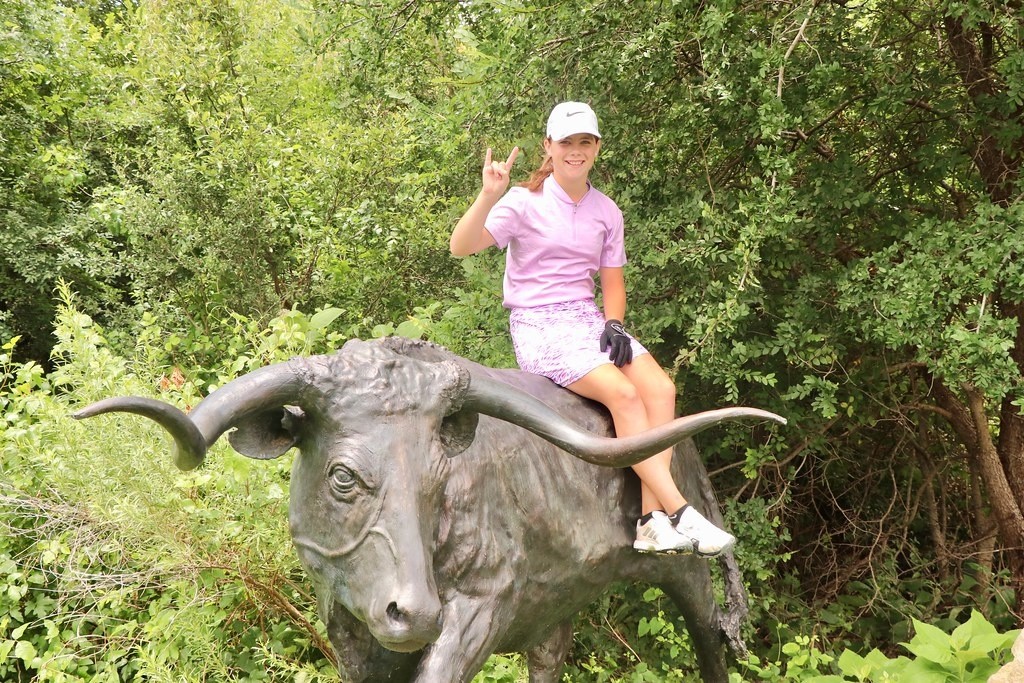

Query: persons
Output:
[449,102,736,560]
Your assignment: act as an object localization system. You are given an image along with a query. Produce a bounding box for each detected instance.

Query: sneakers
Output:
[633,511,692,557]
[669,505,737,558]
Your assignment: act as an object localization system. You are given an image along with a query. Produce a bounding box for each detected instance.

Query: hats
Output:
[547,102,603,141]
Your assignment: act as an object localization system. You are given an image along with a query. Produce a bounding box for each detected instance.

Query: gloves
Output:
[600,320,633,368]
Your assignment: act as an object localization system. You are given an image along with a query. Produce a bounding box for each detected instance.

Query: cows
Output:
[68,335,788,683]
